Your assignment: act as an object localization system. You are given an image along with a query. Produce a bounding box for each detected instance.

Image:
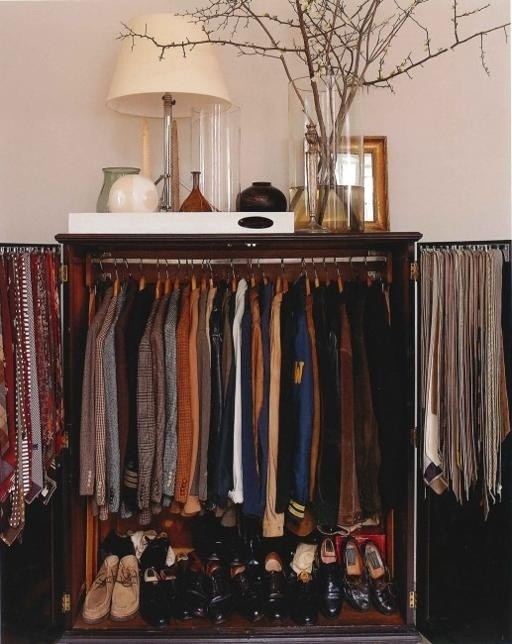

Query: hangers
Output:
[89,258,381,294]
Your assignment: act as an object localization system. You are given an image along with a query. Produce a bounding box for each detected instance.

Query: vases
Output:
[235,181,287,211]
[287,72,367,231]
[96,166,141,213]
[179,170,215,211]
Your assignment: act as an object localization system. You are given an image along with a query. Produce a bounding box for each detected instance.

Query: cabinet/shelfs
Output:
[51,229,433,642]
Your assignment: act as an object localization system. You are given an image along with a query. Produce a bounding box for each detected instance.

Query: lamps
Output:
[107,15,233,213]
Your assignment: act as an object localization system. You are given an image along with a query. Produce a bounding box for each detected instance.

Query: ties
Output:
[0,249,68,548]
[419,247,511,523]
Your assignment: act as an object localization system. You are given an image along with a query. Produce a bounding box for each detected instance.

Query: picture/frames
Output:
[302,135,392,234]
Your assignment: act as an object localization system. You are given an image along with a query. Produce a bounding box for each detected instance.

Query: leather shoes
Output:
[81,528,399,629]
